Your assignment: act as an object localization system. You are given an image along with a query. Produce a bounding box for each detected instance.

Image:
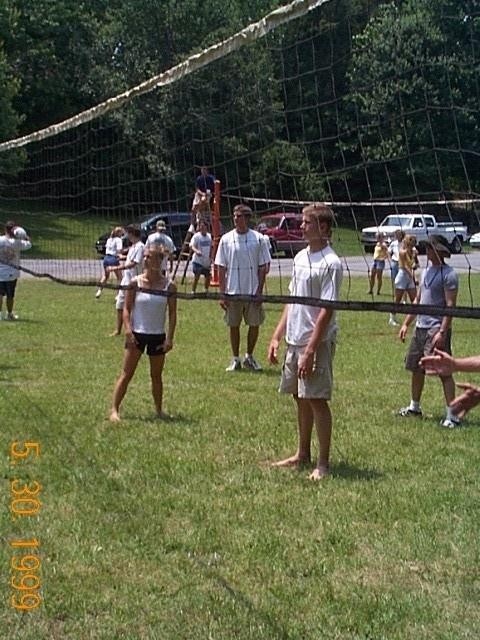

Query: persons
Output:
[145,219,176,273]
[1,220,32,322]
[214,204,271,372]
[365,229,419,326]
[107,244,178,428]
[186,165,217,233]
[266,201,344,481]
[252,216,285,237]
[390,233,464,429]
[108,223,145,336]
[188,220,213,298]
[416,347,480,420]
[95,226,125,297]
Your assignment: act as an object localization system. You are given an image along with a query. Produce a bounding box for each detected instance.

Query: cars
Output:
[469,233,480,249]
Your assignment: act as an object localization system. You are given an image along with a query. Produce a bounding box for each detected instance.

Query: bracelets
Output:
[439,328,447,335]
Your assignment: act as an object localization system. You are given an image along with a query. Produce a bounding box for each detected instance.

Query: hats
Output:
[419,235,451,258]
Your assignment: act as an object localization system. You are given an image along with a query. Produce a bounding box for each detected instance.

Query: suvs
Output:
[256,213,309,260]
[94,210,226,261]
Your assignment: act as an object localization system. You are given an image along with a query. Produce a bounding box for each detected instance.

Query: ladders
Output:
[171,221,198,285]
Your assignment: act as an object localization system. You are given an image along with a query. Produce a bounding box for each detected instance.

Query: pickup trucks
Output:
[360,214,467,258]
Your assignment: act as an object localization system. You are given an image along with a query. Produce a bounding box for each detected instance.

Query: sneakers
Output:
[399,407,422,416]
[243,356,262,371]
[442,419,460,428]
[225,359,241,373]
